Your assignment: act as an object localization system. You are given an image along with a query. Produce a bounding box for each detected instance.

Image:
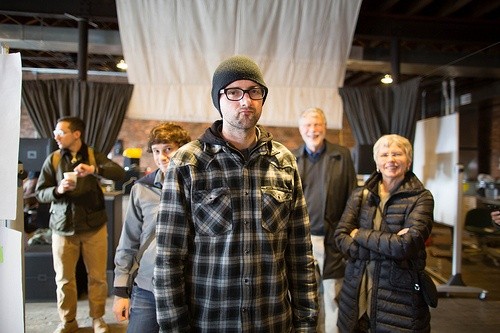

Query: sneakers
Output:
[53,320,79,333]
[93,317,109,333]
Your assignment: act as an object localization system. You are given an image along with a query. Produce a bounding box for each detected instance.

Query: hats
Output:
[211,55,268,118]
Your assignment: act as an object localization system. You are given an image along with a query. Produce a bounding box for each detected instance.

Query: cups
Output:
[62,171,78,191]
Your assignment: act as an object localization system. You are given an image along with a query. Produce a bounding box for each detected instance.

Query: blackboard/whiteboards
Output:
[411,111,461,228]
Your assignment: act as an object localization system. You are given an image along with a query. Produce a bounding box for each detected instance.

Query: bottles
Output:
[484,182,498,202]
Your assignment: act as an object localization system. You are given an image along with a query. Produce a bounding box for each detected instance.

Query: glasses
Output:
[219,87,266,101]
[53,129,76,136]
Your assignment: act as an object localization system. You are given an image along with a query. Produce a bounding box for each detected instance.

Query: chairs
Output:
[462,207,500,271]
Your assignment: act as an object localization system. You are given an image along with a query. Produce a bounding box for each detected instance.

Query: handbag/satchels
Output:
[418,271,439,309]
[125,257,140,297]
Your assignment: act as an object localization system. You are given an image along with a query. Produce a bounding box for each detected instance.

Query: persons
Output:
[111,138,132,169]
[152,54,320,333]
[333,134,439,333]
[34,116,126,333]
[113,120,192,333]
[289,107,358,333]
[23,171,52,233]
